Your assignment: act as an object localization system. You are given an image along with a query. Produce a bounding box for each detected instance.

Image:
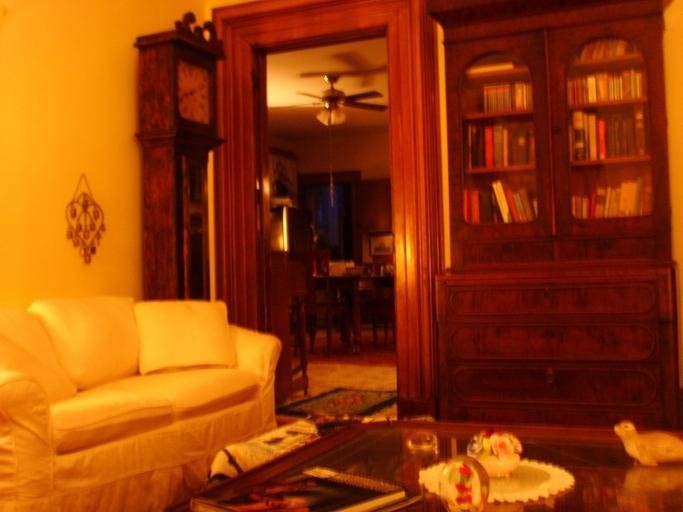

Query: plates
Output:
[419,456,574,505]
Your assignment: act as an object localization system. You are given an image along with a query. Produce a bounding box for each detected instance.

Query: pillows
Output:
[0,335,77,405]
[133,300,236,376]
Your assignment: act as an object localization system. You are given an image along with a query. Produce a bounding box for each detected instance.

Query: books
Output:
[459,31,651,226]
[188,465,409,511]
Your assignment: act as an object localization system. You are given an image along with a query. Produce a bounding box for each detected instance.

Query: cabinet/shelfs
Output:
[423,0,680,432]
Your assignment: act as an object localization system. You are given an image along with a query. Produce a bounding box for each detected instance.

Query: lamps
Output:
[314,106,347,127]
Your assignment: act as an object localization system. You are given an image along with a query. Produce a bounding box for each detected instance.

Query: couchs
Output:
[1,297,282,512]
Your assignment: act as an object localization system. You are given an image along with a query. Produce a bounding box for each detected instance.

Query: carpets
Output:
[273,384,396,425]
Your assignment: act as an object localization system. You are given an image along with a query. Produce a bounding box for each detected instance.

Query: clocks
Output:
[133,10,227,301]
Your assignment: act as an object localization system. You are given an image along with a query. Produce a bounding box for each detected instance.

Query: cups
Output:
[407,428,440,463]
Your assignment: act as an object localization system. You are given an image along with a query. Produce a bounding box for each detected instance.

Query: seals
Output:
[614,421,683,466]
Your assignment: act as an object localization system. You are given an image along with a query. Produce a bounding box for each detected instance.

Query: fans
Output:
[291,75,388,114]
